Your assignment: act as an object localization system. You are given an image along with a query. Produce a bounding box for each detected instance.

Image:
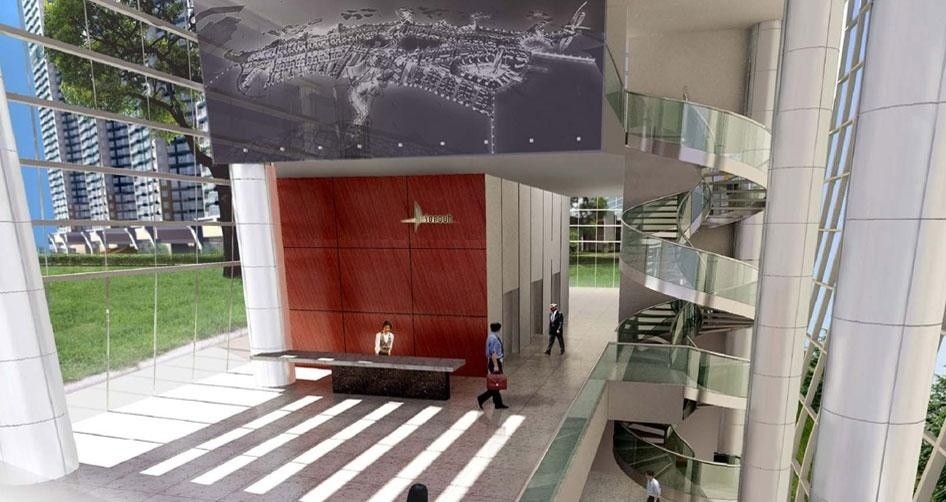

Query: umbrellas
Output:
[476,395,484,411]
[494,404,509,409]
[543,349,565,356]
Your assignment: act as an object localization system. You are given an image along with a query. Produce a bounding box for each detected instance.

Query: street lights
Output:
[486,369,508,391]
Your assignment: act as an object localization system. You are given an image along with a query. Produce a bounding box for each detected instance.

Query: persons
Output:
[645,470,662,502]
[477,323,510,409]
[407,483,428,501]
[374,321,394,356]
[545,303,566,355]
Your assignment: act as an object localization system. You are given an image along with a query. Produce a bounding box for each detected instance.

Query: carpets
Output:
[498,337,505,359]
